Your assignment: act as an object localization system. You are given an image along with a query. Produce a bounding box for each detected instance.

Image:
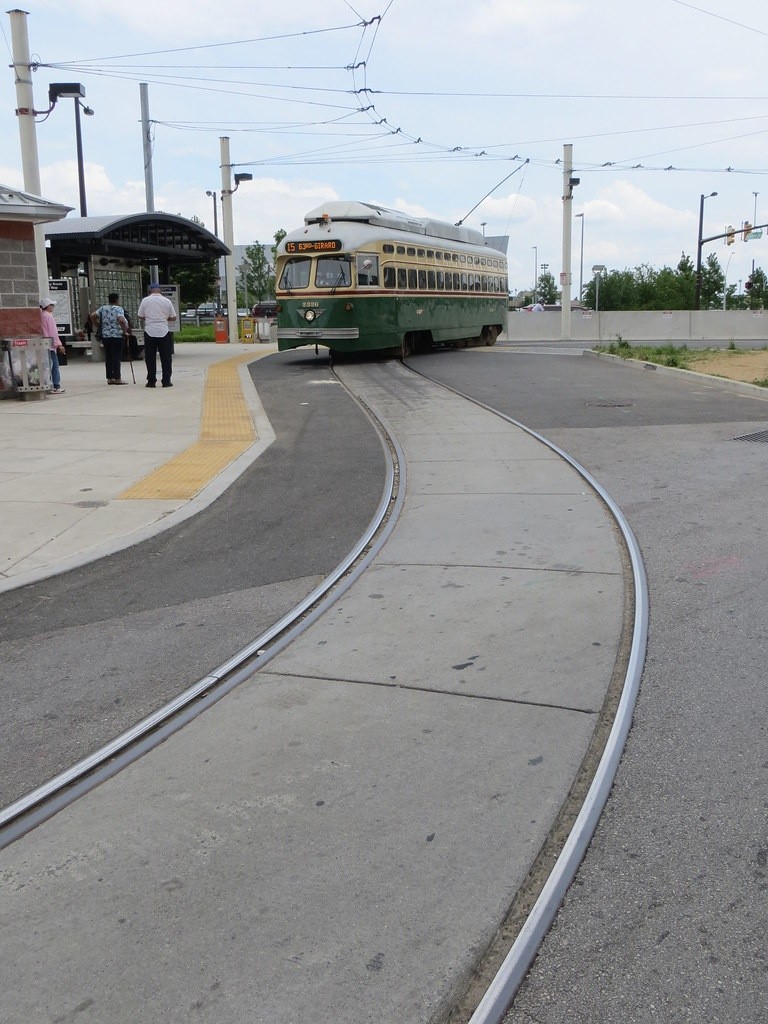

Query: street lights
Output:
[694,192,718,310]
[540,264,549,274]
[561,176,580,338]
[15,82,86,308]
[591,264,605,311]
[532,246,537,303]
[205,191,220,316]
[738,279,742,296]
[480,222,487,238]
[724,251,735,310]
[220,174,253,343]
[575,213,584,307]
[73,98,94,217]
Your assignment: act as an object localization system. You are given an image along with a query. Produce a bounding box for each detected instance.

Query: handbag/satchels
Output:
[57,348,67,366]
[95,325,102,342]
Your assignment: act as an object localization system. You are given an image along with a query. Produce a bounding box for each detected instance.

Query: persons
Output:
[91,293,132,385]
[39,298,65,394]
[138,283,177,387]
[532,297,545,311]
[83,314,93,341]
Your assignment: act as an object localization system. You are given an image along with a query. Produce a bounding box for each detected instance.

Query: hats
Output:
[150,282,160,288]
[39,297,57,309]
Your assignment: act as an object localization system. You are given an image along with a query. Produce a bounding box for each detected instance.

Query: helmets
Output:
[537,297,544,301]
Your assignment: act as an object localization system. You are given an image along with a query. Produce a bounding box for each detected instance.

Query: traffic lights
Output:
[744,221,752,243]
[727,225,735,245]
[745,282,752,288]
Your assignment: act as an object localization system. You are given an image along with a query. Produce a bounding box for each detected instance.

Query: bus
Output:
[275,200,510,361]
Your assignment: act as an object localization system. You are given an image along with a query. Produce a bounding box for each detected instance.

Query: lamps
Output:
[45,239,51,248]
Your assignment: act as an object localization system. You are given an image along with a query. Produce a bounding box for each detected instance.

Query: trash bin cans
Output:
[256,318,270,344]
[0,338,50,401]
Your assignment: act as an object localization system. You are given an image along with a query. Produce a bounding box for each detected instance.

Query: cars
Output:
[183,299,251,318]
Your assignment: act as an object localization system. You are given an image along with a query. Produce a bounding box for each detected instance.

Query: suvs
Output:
[251,301,278,318]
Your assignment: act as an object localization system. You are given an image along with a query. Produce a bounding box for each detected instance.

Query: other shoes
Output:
[50,388,65,393]
[115,378,128,384]
[162,383,173,387]
[107,379,114,384]
[145,383,156,387]
